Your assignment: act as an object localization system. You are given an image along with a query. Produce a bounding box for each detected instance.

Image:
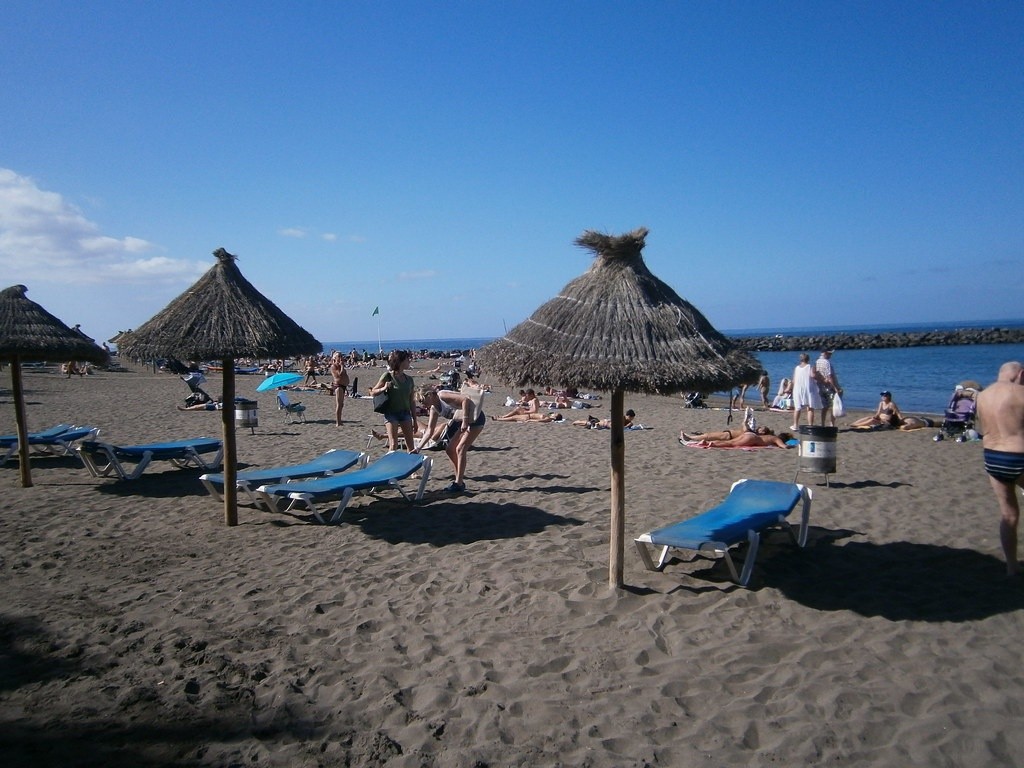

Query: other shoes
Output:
[691,430,703,435]
[444,483,465,493]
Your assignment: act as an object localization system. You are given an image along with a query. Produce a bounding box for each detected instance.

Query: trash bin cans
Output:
[234,397,259,428]
[798,425,837,473]
[265,368,275,377]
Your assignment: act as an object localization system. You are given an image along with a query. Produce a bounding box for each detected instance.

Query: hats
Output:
[820,345,835,353]
[880,391,891,398]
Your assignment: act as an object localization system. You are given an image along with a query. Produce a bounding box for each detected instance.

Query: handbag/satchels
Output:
[832,392,847,417]
[373,370,392,414]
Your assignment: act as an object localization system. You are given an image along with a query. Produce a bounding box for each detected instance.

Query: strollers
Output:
[933,389,978,443]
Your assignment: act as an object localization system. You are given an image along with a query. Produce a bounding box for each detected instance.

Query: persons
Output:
[757,370,770,408]
[409,387,486,493]
[790,353,823,430]
[177,403,215,411]
[371,349,421,479]
[681,426,796,449]
[241,346,485,396]
[330,350,349,426]
[813,347,842,427]
[778,378,792,396]
[849,390,902,430]
[494,385,635,428]
[976,362,1024,576]
[63,360,92,378]
[731,384,748,409]
[889,416,944,429]
[680,390,708,409]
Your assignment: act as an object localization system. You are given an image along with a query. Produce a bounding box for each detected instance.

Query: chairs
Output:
[199,449,372,511]
[73,438,224,480]
[634,478,813,588]
[276,390,307,425]
[235,367,260,375]
[346,376,358,398]
[255,450,434,526]
[367,419,463,450]
[0,422,102,466]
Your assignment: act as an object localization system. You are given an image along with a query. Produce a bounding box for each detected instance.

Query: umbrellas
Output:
[474,228,762,586]
[0,283,111,487]
[110,248,325,527]
[256,372,304,394]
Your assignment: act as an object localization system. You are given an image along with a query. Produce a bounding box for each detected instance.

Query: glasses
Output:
[880,394,889,397]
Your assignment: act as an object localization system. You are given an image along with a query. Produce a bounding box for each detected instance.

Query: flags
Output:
[372,307,378,316]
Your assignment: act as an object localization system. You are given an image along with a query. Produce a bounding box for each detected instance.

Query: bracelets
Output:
[462,416,469,418]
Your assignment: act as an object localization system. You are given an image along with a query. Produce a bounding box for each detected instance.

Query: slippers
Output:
[790,425,800,431]
